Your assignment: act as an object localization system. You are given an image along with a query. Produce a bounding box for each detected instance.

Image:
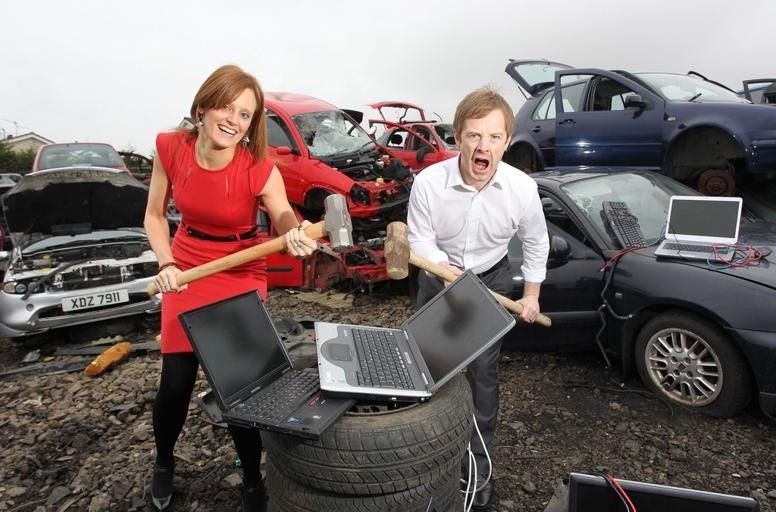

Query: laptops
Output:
[569,472,759,512]
[177,289,357,440]
[655,196,743,263]
[313,269,518,401]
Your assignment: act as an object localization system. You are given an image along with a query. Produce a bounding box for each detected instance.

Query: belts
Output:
[182,221,257,240]
[473,254,508,279]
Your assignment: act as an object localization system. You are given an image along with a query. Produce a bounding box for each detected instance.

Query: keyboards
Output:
[602,201,648,248]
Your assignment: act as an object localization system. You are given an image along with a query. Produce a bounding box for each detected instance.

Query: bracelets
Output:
[154,261,183,275]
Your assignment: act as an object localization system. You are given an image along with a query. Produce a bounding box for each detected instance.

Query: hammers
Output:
[148,194,355,297]
[385,222,552,327]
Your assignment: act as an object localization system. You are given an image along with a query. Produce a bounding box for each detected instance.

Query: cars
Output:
[502,57,776,203]
[257,91,462,296]
[0,167,163,343]
[33,142,154,185]
[497,167,775,420]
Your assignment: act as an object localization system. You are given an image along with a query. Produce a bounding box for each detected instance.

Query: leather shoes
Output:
[467,468,494,509]
[150,459,175,510]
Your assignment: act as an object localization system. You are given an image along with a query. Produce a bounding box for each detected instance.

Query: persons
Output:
[407,89,551,511]
[141,63,318,509]
